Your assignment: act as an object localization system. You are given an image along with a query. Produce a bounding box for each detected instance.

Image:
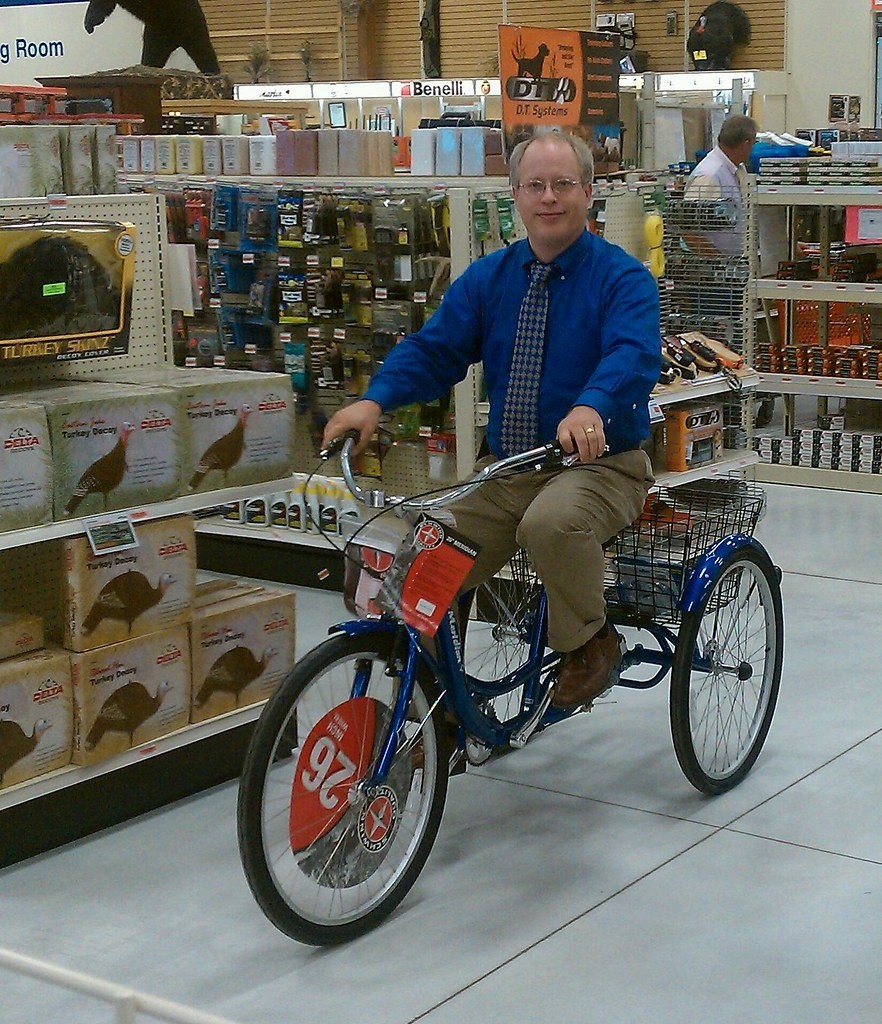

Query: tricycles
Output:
[237,425,790,945]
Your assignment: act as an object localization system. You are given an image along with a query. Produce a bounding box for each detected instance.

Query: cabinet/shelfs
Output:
[477,372,760,630]
[0,474,299,871]
[740,174,882,495]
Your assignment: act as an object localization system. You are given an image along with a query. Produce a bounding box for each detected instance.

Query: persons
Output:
[671,113,758,350]
[323,127,664,708]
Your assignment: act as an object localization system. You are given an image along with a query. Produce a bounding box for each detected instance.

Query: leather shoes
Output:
[408,711,461,769]
[552,621,620,712]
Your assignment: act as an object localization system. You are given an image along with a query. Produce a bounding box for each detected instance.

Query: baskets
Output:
[509,483,762,630]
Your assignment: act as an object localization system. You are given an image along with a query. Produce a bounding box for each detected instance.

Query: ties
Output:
[500,261,555,459]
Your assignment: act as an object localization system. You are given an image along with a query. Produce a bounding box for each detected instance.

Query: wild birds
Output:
[185,401,257,493]
[0,717,56,790]
[192,643,282,712]
[78,567,181,640]
[61,420,139,519]
[82,679,176,755]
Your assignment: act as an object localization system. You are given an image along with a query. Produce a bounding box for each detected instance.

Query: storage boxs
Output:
[754,342,882,387]
[0,513,296,790]
[0,364,294,534]
[777,241,882,290]
[756,157,882,186]
[0,219,138,368]
[795,128,841,151]
[0,125,117,198]
[654,402,724,472]
[745,414,882,475]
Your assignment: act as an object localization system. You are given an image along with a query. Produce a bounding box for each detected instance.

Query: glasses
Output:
[518,178,585,197]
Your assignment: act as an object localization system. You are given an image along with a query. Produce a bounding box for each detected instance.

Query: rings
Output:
[586,428,594,433]
